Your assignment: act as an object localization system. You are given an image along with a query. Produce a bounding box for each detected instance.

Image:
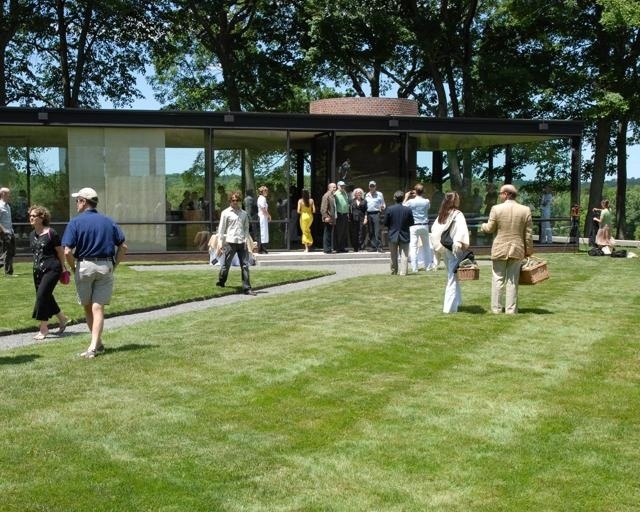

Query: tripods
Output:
[563,217,589,256]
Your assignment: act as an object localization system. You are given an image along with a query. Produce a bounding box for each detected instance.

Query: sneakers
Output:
[258,244,269,254]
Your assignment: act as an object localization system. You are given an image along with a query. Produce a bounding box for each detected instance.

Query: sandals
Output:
[95,345,105,353]
[77,349,97,359]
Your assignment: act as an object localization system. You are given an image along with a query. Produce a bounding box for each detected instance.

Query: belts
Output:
[77,257,113,261]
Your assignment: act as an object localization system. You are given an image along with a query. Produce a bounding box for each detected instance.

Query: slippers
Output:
[33,328,49,340]
[58,316,69,333]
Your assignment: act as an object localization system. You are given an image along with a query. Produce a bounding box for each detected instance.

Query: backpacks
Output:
[587,247,607,256]
[611,250,627,257]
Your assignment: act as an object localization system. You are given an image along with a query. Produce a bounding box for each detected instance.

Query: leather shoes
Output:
[216,282,225,288]
[243,288,257,295]
[303,243,384,253]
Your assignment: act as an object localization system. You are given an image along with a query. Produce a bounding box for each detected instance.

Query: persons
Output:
[165,186,255,221]
[430,190,469,313]
[0,187,19,278]
[257,181,386,254]
[380,183,433,276]
[60,188,128,359]
[12,190,28,232]
[470,187,484,244]
[481,181,552,315]
[27,205,70,340]
[592,199,612,250]
[216,192,257,297]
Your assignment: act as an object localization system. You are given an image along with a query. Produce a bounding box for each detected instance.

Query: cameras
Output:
[410,192,414,194]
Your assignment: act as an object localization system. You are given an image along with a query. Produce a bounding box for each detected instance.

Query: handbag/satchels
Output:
[440,211,461,251]
[60,271,73,284]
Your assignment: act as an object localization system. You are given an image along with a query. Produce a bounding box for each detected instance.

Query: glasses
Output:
[232,200,241,202]
[29,215,40,217]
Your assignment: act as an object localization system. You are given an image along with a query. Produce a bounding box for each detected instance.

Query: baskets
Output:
[519,255,552,285]
[457,268,480,280]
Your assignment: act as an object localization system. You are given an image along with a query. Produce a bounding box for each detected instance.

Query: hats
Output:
[340,182,346,186]
[369,180,376,186]
[71,187,99,203]
